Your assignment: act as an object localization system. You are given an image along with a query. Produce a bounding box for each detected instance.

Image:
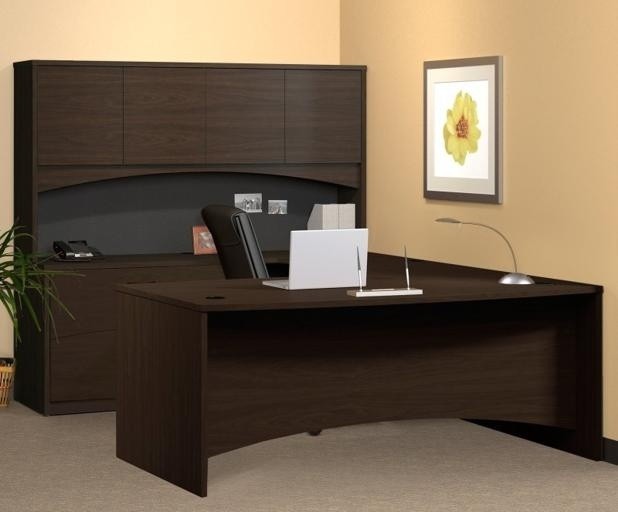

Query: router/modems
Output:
[346,246,423,297]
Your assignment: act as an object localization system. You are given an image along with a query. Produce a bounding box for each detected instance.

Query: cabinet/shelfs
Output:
[14,60,367,416]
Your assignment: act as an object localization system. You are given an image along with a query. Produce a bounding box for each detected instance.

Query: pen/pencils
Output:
[356,244,364,289]
[404,245,410,290]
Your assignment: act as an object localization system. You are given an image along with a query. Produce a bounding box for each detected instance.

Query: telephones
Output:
[53,240,105,262]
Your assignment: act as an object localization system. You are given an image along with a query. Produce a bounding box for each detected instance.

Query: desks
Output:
[116,275,604,497]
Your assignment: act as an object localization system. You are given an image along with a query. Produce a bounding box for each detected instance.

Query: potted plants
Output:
[0,216,88,408]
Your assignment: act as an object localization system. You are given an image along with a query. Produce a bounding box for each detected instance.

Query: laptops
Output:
[262,228,368,291]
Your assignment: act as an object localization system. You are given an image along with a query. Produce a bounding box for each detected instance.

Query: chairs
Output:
[201,204,270,279]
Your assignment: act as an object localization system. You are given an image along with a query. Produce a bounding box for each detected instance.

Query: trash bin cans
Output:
[0,357,17,407]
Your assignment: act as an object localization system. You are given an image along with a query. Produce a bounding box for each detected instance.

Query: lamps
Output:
[435,218,535,285]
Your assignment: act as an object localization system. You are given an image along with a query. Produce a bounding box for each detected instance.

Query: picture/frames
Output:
[423,56,504,205]
[192,225,218,255]
